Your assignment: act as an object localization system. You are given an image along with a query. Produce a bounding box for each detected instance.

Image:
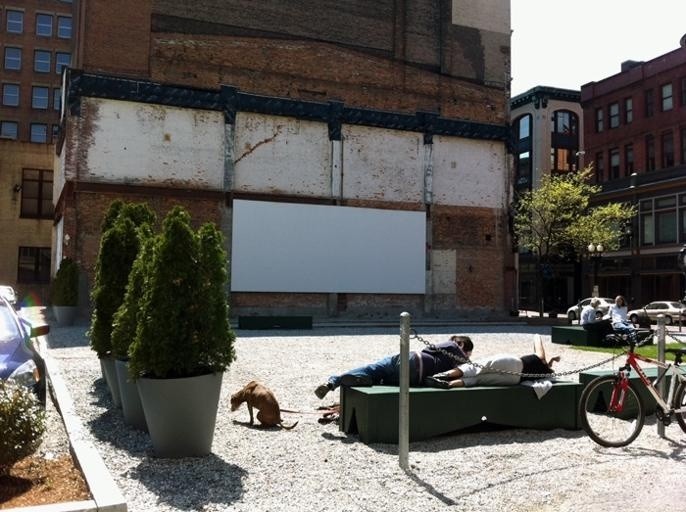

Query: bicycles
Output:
[578,321,686,449]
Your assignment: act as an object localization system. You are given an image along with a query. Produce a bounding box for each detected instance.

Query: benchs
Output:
[338,377,584,444]
[580,364,685,414]
[551,326,655,349]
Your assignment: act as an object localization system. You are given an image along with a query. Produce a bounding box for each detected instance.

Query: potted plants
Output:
[86,199,237,457]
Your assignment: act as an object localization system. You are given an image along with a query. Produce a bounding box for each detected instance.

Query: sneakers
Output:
[315,382,331,399]
[425,373,449,388]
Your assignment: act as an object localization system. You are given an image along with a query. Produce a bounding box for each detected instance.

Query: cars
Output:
[0,285,18,311]
[626,300,686,326]
[0,293,53,417]
[566,296,628,321]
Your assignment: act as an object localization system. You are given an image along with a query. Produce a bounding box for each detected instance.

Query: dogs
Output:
[229,380,299,431]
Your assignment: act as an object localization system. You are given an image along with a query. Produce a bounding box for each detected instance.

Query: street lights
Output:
[588,242,605,297]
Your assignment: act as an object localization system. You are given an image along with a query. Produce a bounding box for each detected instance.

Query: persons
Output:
[581,297,610,345]
[425,333,561,389]
[314,335,474,400]
[608,295,639,347]
